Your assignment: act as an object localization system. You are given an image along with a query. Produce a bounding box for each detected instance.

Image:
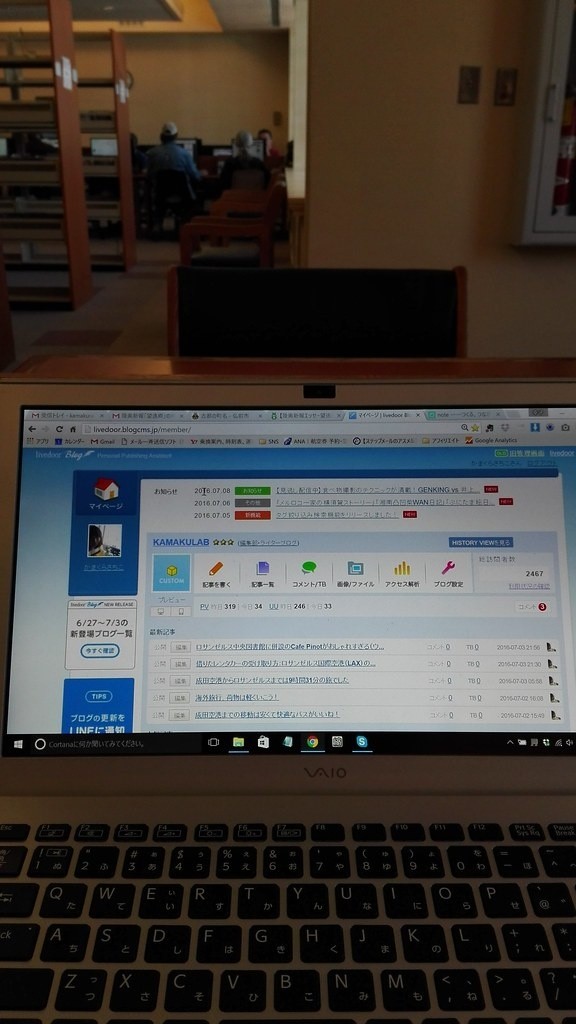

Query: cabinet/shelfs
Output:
[0,0,133,312]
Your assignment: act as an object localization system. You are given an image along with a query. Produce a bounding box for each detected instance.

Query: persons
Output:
[143,122,200,202]
[218,131,270,189]
[130,133,145,173]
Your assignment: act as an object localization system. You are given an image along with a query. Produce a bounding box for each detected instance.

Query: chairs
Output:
[165,264,466,357]
[136,175,280,266]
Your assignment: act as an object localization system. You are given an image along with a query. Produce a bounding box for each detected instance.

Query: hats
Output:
[235,131,252,147]
[162,122,178,135]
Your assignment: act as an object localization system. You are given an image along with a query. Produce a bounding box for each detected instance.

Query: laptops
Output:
[0,376,576,1024]
